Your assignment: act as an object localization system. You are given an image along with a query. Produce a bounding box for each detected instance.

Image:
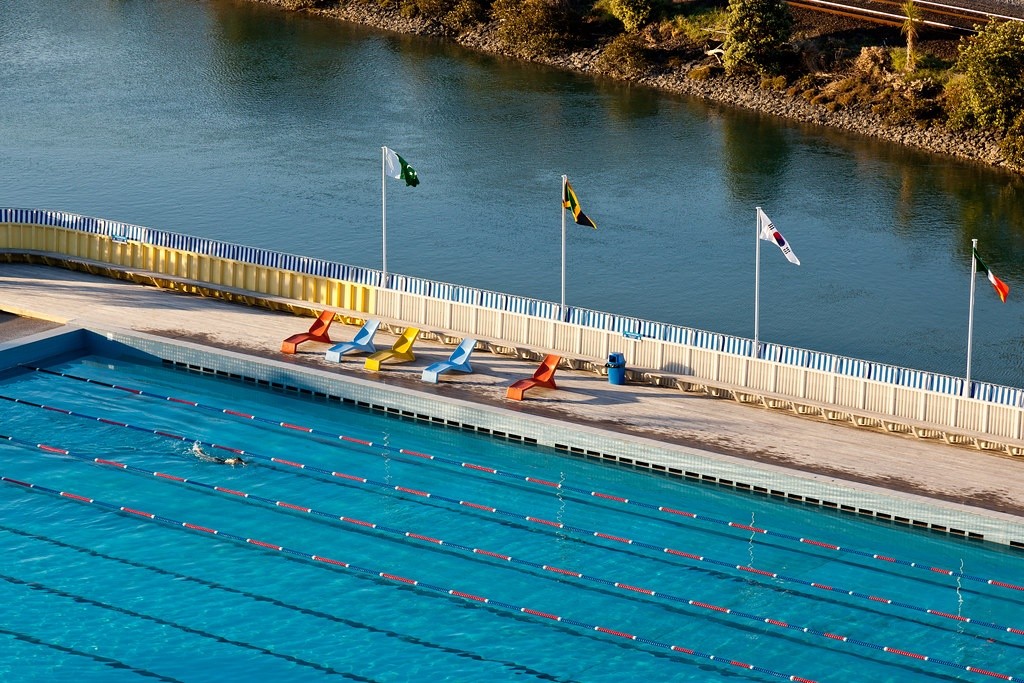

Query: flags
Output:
[974,251,1009,303]
[386,146,420,187]
[564,179,596,229]
[759,208,800,266]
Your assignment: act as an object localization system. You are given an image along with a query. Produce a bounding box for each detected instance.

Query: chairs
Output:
[421,337,477,383]
[506,354,562,401]
[281,309,336,353]
[326,319,380,362]
[364,326,420,371]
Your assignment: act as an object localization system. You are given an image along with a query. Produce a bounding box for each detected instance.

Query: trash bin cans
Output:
[605,352,626,385]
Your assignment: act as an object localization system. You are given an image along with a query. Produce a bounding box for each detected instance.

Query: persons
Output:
[194,444,243,463]
